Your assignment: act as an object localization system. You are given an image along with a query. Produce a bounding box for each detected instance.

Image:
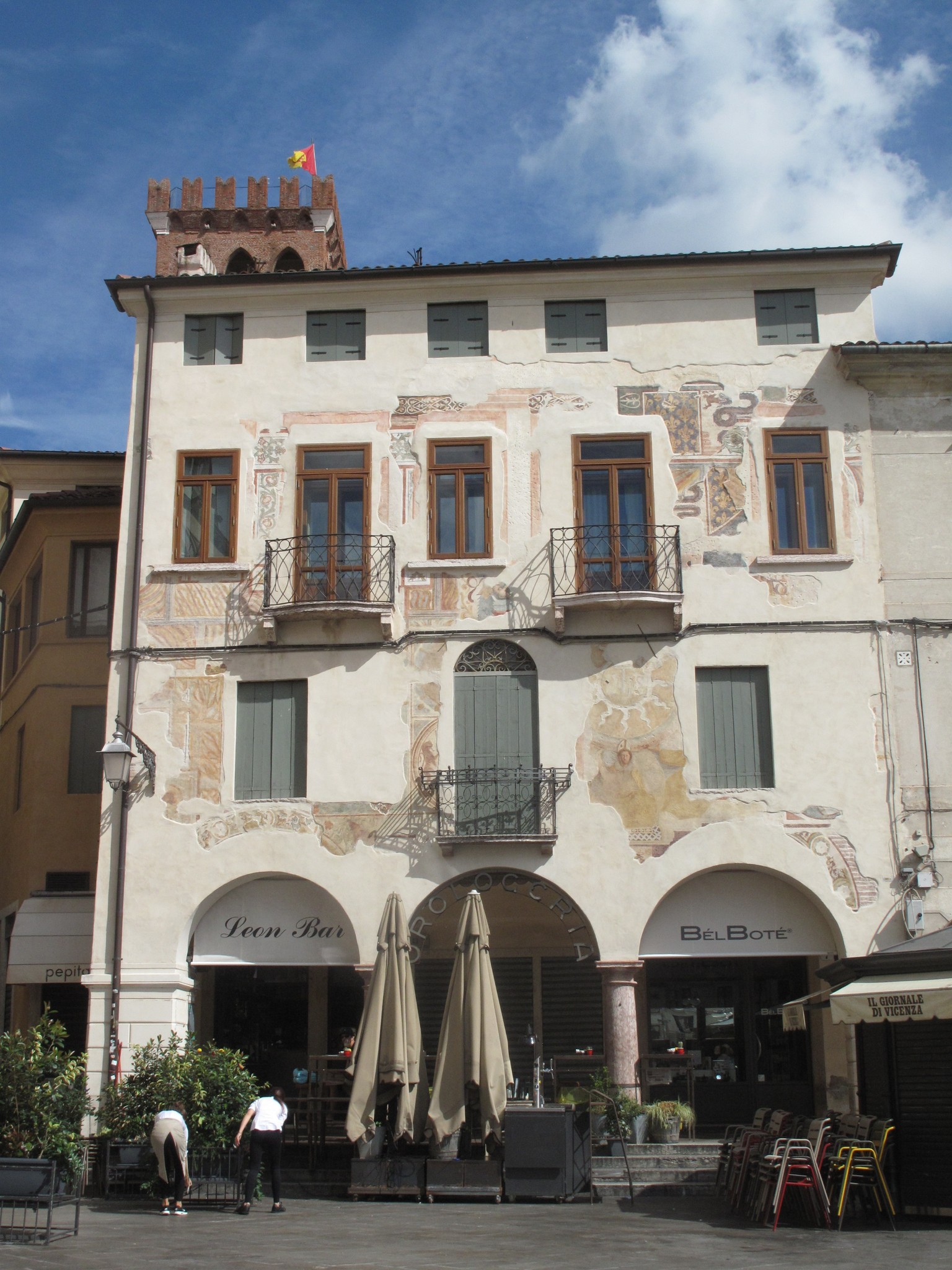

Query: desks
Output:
[639,1053,697,1141]
[552,1055,605,1104]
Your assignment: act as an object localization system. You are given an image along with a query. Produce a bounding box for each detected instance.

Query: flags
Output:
[287,144,317,176]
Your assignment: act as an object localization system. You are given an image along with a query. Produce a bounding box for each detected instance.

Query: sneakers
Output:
[174,1207,188,1215]
[160,1206,171,1215]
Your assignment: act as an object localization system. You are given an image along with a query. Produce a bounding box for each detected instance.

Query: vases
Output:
[428,1127,461,1159]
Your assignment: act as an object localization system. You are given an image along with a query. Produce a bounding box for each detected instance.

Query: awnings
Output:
[782,978,855,1031]
[830,970,952,1025]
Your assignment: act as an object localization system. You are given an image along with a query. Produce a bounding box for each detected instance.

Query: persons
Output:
[235,1087,288,1215]
[151,1110,193,1215]
[341,1027,357,1050]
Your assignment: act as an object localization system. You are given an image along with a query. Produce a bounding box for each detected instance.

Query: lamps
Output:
[94,710,156,794]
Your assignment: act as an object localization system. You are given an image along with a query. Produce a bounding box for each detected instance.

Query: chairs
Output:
[715,1107,896,1233]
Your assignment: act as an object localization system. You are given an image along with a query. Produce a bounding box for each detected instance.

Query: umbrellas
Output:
[345,891,430,1146]
[423,889,515,1148]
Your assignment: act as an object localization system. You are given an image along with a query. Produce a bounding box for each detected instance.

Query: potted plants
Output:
[586,1047,594,1055]
[677,1041,684,1054]
[357,1121,386,1159]
[0,998,270,1196]
[585,1065,697,1144]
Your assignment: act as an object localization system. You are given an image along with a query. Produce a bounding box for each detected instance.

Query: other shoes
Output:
[237,1202,250,1214]
[271,1201,286,1212]
